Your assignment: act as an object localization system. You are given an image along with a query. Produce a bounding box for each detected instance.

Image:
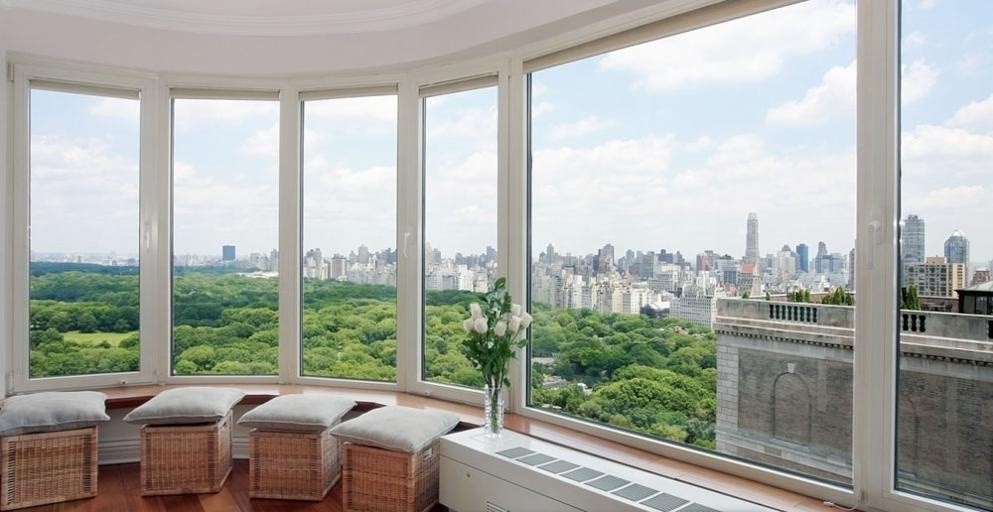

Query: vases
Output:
[482,380,505,437]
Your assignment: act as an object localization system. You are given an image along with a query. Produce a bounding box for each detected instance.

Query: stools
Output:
[138,411,232,497]
[0,423,98,510]
[248,424,342,500]
[341,439,440,512]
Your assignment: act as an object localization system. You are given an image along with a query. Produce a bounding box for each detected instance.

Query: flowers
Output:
[457,276,533,432]
